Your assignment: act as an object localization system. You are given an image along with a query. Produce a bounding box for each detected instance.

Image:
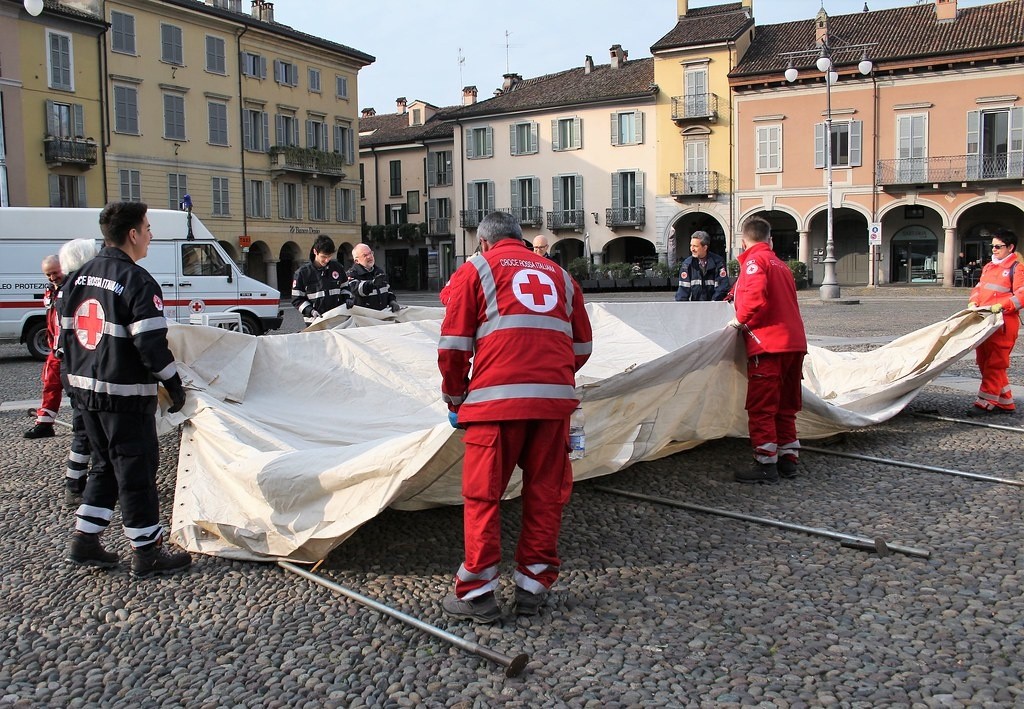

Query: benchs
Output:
[930,269,944,283]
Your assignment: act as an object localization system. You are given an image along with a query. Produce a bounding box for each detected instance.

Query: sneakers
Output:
[735,459,779,484]
[991,405,1016,415]
[65,531,118,567]
[516,586,544,614]
[130,534,191,578]
[967,404,992,418]
[23,424,55,438]
[64,490,83,506]
[443,596,501,624]
[776,457,801,477]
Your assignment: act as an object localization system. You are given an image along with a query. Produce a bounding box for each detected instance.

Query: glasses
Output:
[989,244,1012,249]
[361,252,374,259]
[534,244,548,249]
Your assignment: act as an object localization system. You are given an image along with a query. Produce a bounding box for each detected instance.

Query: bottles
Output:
[567,407,586,460]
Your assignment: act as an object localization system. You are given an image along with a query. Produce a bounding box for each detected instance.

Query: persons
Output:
[63,200,194,578]
[967,229,1024,416]
[292,235,354,328]
[957,252,980,276]
[437,212,593,625]
[533,234,552,260]
[675,231,734,302]
[724,217,808,486]
[340,244,400,313]
[24,255,69,438]
[59,239,99,503]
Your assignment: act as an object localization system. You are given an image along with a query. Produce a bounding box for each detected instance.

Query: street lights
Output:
[777,41,877,304]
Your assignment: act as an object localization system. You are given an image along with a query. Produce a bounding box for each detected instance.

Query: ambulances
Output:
[0,193,286,363]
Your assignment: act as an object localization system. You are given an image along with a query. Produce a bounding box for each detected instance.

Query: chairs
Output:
[954,269,968,288]
[969,268,982,288]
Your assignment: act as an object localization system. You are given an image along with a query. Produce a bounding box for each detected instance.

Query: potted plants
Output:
[786,260,808,290]
[727,260,740,287]
[567,257,682,288]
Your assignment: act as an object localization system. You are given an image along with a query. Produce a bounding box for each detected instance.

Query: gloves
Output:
[968,302,976,308]
[449,412,463,430]
[390,301,401,313]
[312,310,323,320]
[728,318,745,335]
[346,298,355,309]
[162,373,186,413]
[370,274,388,288]
[990,304,1002,313]
